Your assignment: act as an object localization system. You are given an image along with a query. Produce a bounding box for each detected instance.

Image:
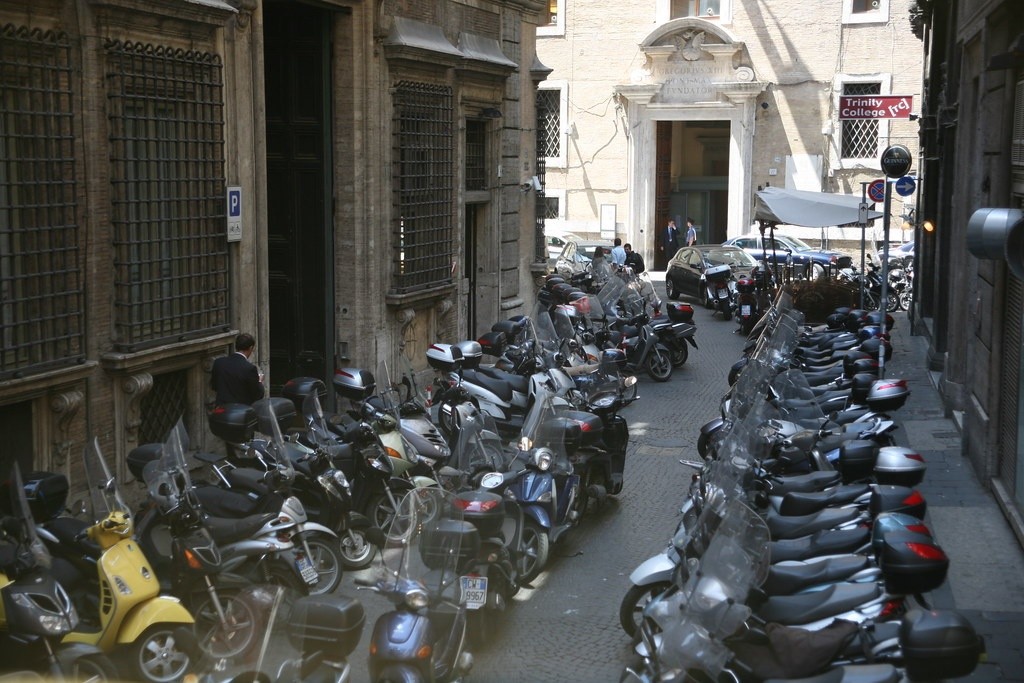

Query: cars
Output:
[544,231,585,259]
[555,241,615,278]
[666,244,766,309]
[877,242,914,263]
[720,234,852,283]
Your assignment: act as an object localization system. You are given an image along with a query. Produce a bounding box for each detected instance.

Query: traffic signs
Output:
[838,94,914,120]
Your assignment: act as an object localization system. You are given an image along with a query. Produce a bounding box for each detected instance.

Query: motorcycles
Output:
[0,253,987,683]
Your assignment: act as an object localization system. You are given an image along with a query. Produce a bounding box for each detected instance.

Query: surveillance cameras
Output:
[531,176,542,192]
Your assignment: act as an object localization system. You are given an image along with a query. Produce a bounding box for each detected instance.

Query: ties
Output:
[669,228,671,239]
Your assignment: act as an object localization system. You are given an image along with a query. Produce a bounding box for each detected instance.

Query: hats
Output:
[687,216,695,225]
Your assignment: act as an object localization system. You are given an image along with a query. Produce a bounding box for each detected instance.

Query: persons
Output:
[660,217,697,268]
[209,334,265,468]
[593,238,645,276]
